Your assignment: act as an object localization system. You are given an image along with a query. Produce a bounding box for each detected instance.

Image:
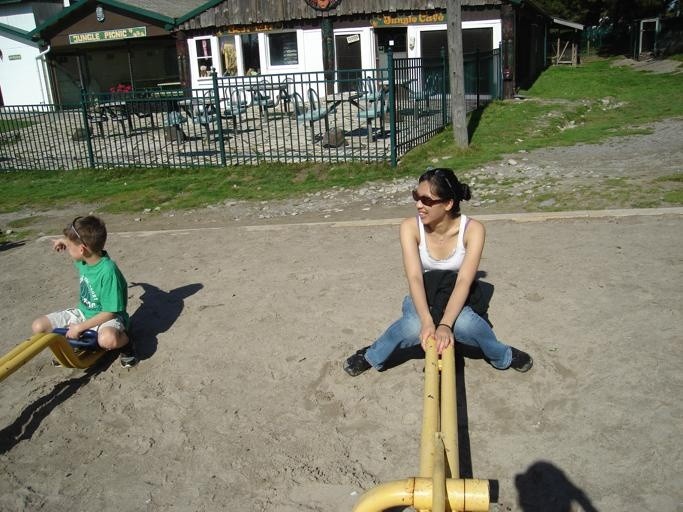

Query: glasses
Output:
[427,167,452,188]
[71,216,86,247]
[412,190,445,206]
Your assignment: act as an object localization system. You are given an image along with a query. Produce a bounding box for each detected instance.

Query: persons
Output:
[32,215,139,368]
[343,168,533,376]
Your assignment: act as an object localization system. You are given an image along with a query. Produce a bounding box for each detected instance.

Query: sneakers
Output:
[509,346,533,372]
[343,346,371,376]
[51,347,79,367]
[119,331,138,368]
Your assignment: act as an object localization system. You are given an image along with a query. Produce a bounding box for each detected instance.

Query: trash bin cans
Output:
[504,69,513,100]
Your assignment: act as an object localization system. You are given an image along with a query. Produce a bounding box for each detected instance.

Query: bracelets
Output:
[437,324,453,331]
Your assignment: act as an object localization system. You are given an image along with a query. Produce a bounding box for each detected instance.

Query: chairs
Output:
[85,71,442,144]
